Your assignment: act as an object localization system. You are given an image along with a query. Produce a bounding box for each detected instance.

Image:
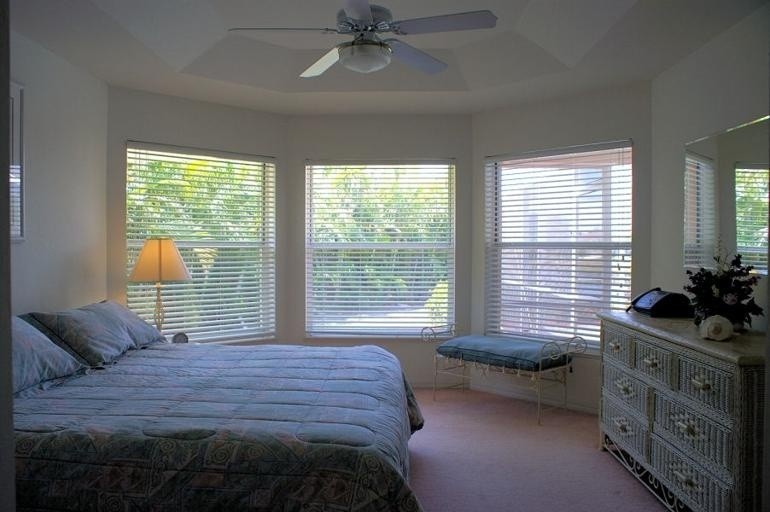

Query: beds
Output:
[13,342,413,512]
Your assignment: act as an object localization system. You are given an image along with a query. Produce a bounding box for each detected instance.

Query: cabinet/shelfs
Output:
[599,319,770,512]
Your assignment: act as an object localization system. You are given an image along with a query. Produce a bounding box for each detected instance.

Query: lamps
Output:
[127,235,194,334]
[337,4,395,74]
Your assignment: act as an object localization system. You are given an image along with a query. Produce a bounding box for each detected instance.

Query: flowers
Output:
[682,231,766,328]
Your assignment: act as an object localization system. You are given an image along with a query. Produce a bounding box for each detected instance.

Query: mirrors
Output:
[684,114,770,276]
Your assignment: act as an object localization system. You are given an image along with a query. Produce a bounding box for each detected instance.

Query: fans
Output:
[227,0,500,78]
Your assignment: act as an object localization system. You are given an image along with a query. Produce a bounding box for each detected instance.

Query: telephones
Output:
[631,288,690,317]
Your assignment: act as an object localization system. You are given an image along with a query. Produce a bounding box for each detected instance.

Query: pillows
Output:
[10,300,167,400]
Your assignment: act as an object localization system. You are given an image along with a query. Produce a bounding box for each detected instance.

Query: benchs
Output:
[420,323,587,426]
